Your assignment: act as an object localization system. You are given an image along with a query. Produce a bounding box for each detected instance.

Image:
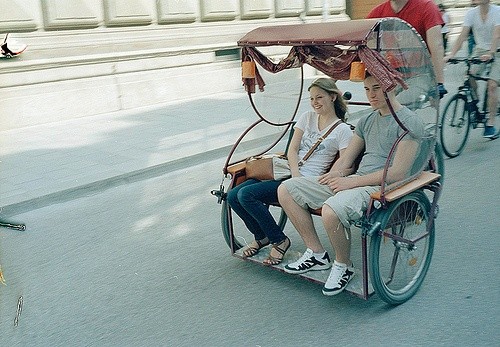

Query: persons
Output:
[439,4,449,57]
[277,71,424,296]
[226,77,353,266]
[367,0,446,99]
[439,0,500,137]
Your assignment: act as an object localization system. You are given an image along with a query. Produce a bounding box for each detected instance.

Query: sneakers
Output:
[321,260,356,296]
[284,248,331,274]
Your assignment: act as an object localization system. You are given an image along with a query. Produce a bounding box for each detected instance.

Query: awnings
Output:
[237,17,439,196]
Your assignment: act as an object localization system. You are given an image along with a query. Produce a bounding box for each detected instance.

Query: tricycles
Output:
[210,16,447,304]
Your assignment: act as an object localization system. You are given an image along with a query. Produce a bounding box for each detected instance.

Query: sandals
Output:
[263,236,291,265]
[243,237,271,257]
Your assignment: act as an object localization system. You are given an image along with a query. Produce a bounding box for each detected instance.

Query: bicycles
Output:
[440,57,500,157]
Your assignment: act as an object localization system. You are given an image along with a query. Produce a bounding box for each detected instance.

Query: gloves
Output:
[428,83,447,99]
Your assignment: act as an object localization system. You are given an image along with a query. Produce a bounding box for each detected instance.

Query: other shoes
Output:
[482,126,496,138]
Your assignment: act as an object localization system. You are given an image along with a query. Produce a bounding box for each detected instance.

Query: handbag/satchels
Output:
[272,157,291,182]
